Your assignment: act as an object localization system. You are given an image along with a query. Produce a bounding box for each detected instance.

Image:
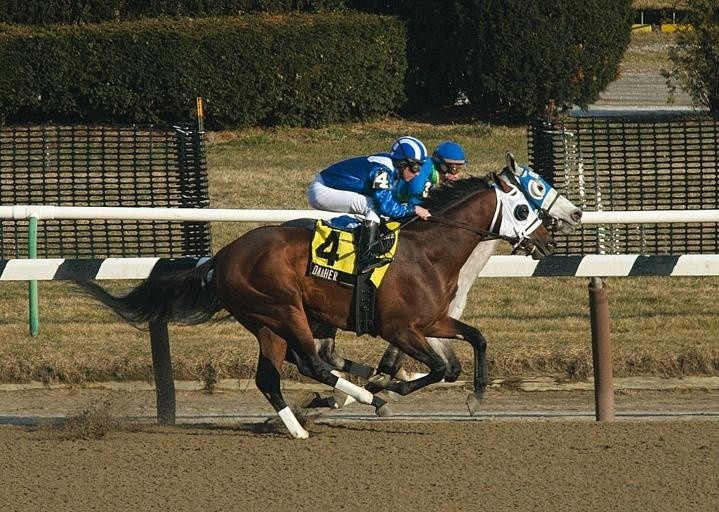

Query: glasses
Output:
[396,140,419,173]
[433,151,461,174]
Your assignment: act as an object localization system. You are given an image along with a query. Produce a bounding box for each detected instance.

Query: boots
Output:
[358,219,393,274]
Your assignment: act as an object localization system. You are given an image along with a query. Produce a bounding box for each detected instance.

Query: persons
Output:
[307,135,432,274]
[392,141,466,205]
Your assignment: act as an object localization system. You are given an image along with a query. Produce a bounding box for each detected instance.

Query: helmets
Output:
[390,136,427,165]
[433,142,466,164]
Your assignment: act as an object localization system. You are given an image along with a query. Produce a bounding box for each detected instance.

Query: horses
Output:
[202,149,585,417]
[60,171,558,441]
